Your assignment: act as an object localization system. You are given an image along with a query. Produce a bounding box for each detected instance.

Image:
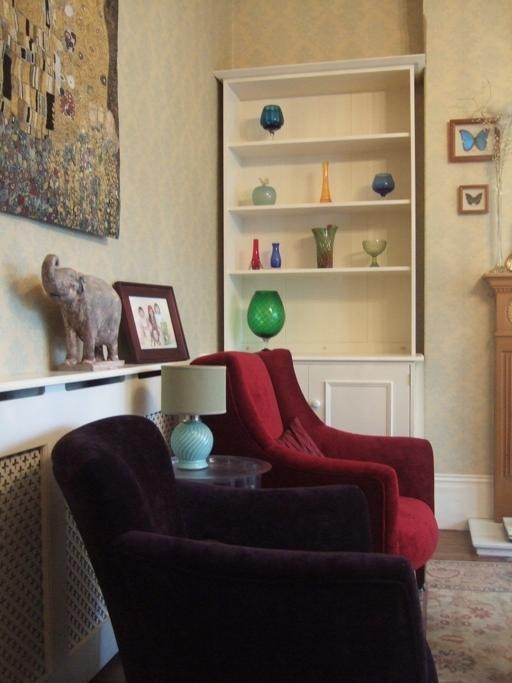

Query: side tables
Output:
[171,455,272,489]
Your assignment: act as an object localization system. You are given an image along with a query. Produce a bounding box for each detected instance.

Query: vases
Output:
[319,160,333,204]
[490,233,508,273]
[312,225,337,269]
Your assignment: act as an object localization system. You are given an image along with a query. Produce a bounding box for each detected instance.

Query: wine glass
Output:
[260,105,285,140]
[247,290,286,351]
[371,173,394,200]
[363,239,387,267]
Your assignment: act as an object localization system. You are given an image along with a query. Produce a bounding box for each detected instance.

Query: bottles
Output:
[248,235,262,270]
[270,243,281,268]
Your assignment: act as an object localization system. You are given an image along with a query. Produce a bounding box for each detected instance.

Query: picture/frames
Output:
[114,280,191,364]
[450,118,500,162]
[459,184,489,214]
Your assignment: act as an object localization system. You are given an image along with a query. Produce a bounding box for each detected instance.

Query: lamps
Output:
[161,366,228,471]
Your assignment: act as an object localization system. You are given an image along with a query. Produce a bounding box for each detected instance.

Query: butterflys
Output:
[465,192,483,206]
[459,128,490,152]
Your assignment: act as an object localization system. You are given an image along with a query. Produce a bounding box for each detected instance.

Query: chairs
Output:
[51,415,440,681]
[191,348,439,589]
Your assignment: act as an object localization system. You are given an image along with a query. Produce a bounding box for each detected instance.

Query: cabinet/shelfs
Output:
[294,361,424,438]
[216,52,416,356]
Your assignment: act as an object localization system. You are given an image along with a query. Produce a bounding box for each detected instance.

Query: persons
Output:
[137,300,172,349]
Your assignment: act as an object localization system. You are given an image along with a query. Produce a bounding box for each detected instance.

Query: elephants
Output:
[41,253,122,365]
